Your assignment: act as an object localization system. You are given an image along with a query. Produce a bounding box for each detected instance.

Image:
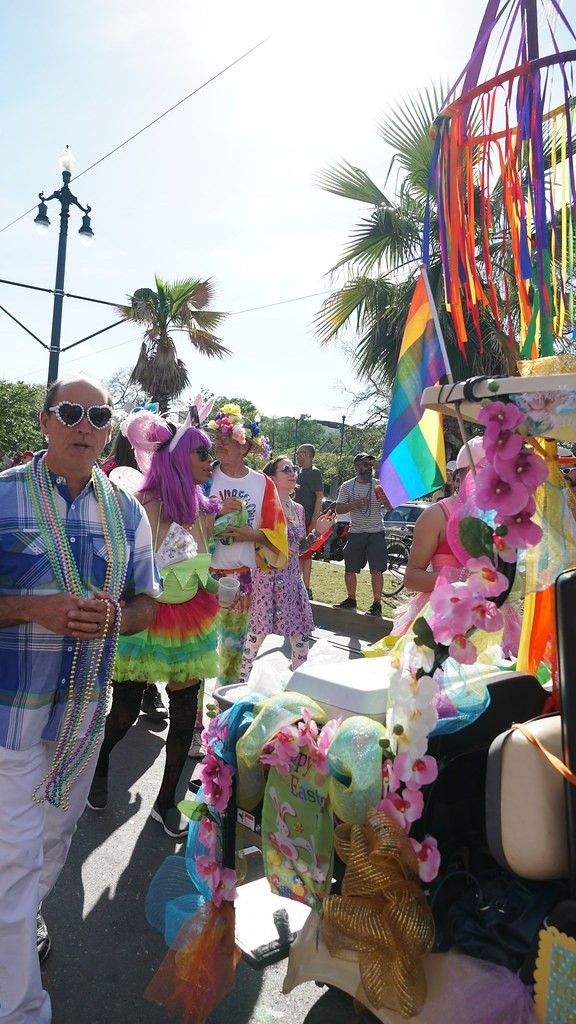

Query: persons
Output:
[0,378,163,1024]
[391,437,486,635]
[89,403,338,837]
[335,452,392,615]
[290,444,323,600]
[0,449,33,468]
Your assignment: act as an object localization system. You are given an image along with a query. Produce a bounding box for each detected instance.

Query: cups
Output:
[219,577,240,608]
[374,485,383,501]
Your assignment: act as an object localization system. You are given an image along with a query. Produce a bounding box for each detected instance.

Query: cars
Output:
[318,497,336,516]
[381,500,436,562]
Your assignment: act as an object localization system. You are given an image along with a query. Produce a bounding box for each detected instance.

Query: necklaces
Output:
[25,449,128,813]
[183,516,197,531]
[280,498,290,507]
[353,478,372,517]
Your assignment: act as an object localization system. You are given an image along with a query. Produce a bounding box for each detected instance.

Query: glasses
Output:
[192,447,208,461]
[275,465,300,474]
[358,462,373,468]
[560,467,576,474]
[49,401,114,430]
[21,456,26,459]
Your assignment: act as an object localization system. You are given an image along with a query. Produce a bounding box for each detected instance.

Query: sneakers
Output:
[87,762,108,810]
[188,733,206,757]
[150,801,189,838]
[141,687,167,719]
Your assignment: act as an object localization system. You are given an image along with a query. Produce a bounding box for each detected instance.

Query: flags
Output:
[380,276,449,508]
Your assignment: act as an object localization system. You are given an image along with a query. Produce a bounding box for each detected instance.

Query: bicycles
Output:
[379,531,413,598]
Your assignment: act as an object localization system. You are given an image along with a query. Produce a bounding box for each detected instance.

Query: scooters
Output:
[309,524,351,562]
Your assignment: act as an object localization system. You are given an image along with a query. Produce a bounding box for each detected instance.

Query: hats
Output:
[203,415,262,454]
[456,436,485,469]
[354,453,375,461]
[557,446,575,466]
[24,451,34,456]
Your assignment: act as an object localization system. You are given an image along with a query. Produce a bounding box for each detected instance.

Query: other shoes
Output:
[37,910,50,962]
[312,556,319,560]
[334,598,356,608]
[324,558,330,563]
[366,602,381,616]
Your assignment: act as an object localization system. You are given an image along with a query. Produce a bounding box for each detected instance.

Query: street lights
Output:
[269,410,278,450]
[253,412,261,469]
[34,142,96,449]
[339,408,348,460]
[293,412,301,466]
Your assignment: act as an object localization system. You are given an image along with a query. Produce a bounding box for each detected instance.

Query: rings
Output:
[361,500,364,502]
